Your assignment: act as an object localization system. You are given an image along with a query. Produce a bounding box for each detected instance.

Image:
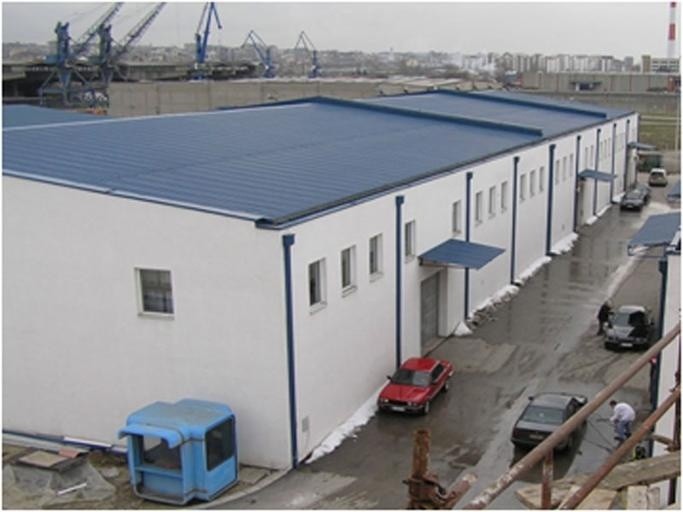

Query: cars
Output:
[506,391,588,453]
[601,302,656,355]
[374,354,454,417]
[620,187,649,213]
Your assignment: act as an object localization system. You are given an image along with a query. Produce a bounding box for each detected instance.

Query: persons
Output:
[610,399,636,443]
[597,301,612,335]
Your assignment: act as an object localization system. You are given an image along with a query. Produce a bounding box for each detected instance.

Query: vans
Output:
[649,168,667,185]
